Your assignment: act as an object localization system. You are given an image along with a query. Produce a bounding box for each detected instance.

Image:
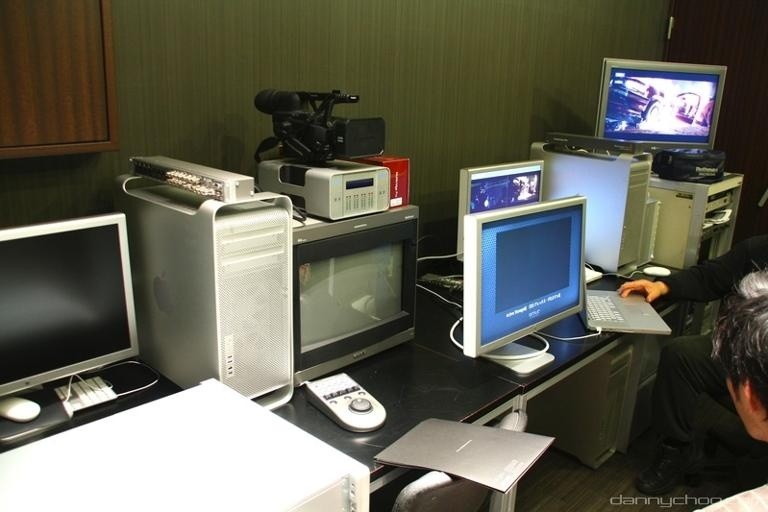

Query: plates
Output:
[0,1,120,158]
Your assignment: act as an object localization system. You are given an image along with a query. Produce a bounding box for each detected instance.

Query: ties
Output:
[457,160,544,263]
[0,207,140,397]
[595,56,727,152]
[280,204,420,390]
[463,195,586,375]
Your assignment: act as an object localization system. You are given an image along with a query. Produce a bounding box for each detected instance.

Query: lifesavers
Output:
[528,140,653,277]
[528,341,637,468]
[114,156,293,418]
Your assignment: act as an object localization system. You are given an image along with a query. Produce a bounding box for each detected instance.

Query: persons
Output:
[695,271,768,512]
[618,234,768,495]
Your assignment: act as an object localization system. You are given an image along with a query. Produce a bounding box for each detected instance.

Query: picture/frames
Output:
[0,340,523,511]
[414,240,686,471]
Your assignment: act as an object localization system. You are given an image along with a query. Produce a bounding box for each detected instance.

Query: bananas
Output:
[585,265,603,286]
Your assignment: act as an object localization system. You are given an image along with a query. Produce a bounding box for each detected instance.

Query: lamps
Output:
[392,408,530,511]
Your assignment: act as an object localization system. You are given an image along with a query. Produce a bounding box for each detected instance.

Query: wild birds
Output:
[578,285,672,336]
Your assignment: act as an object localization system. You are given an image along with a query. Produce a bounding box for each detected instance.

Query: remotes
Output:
[0,396,42,422]
[643,266,671,278]
